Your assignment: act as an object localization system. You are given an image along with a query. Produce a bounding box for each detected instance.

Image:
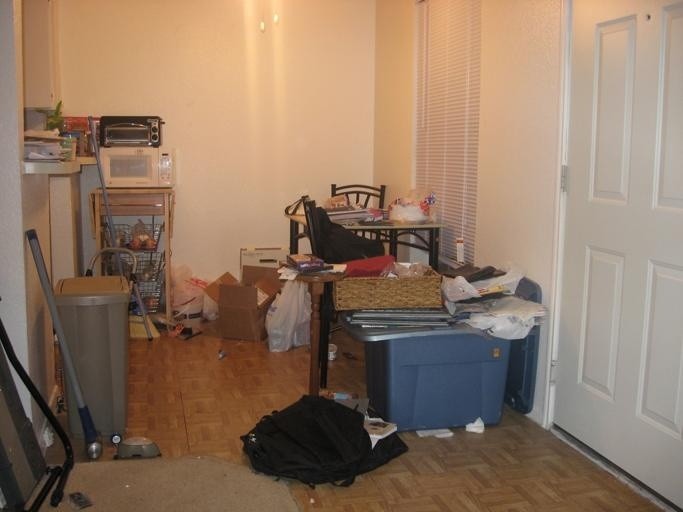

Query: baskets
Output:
[104,223,165,313]
[332,266,443,311]
[390,219,429,225]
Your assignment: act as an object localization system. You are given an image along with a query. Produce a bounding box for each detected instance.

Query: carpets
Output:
[24,454,300,512]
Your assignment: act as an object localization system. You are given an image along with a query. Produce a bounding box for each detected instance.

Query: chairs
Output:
[330,183,386,242]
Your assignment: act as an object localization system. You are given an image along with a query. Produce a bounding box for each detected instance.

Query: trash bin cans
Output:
[51,275,131,445]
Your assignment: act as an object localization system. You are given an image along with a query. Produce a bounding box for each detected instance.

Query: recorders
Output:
[99,115,161,146]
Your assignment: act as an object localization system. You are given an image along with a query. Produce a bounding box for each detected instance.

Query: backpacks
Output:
[240,395,409,489]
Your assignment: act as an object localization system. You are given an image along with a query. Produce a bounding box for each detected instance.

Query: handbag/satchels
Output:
[285,196,385,263]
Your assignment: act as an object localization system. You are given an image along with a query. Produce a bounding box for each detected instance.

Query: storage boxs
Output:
[204,265,284,341]
[338,311,511,433]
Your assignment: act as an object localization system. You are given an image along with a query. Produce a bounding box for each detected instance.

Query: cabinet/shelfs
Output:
[88,187,173,333]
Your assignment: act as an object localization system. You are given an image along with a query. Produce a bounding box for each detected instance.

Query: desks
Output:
[279,260,379,397]
[284,213,444,270]
